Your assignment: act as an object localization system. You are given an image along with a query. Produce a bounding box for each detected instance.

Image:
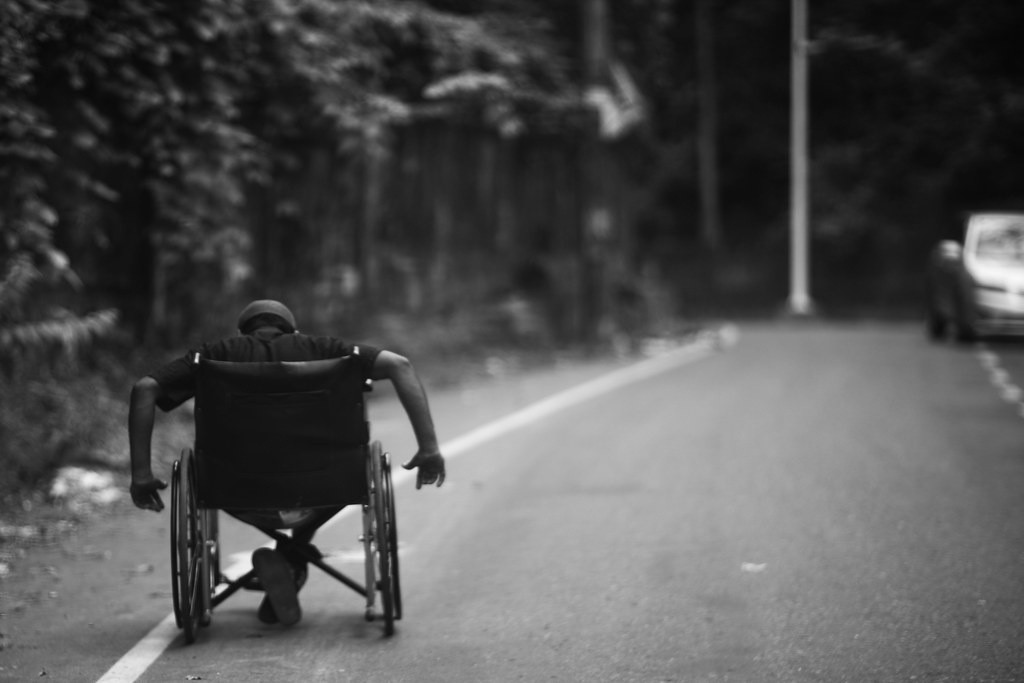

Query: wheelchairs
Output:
[170,350,403,644]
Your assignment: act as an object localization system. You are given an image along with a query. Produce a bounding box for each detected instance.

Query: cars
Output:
[917,212,1024,345]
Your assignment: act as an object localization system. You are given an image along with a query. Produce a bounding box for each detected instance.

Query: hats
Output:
[237,300,298,333]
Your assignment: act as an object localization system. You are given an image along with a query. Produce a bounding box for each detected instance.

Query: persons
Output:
[127,299,447,627]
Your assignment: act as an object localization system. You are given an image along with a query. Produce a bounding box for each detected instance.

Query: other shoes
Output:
[252,547,301,627]
[257,564,310,624]
